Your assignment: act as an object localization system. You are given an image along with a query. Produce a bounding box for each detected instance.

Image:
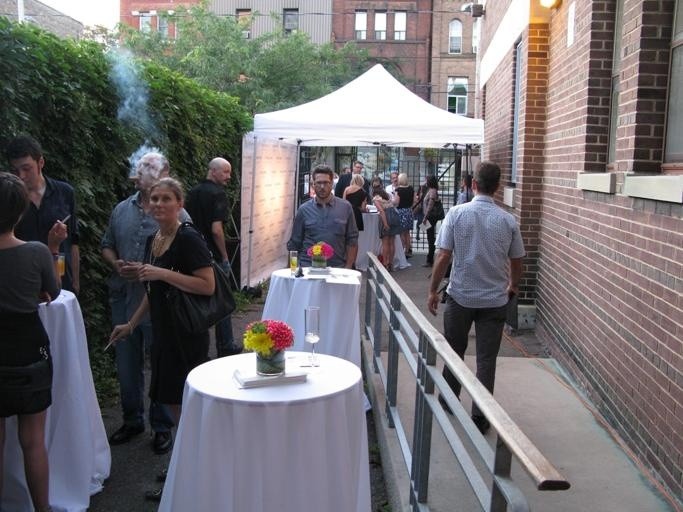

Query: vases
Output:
[312,256,325,268]
[254,349,286,376]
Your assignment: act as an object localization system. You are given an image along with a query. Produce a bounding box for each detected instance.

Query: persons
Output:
[426,160,525,432]
[0,171,70,511]
[106,151,195,456]
[286,161,475,271]
[108,177,218,499]
[5,133,81,299]
[184,157,245,356]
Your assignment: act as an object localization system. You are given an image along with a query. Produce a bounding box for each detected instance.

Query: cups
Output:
[56,253,65,275]
[289,249,298,276]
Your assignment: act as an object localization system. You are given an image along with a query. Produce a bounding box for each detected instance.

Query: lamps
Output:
[539,0,565,10]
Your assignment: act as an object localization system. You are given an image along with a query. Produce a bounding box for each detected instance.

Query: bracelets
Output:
[51,252,59,262]
[128,320,134,334]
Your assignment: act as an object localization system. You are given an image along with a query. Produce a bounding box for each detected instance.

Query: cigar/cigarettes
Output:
[104,341,113,351]
[62,214,72,223]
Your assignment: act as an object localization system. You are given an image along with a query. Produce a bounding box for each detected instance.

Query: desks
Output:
[0,287,114,512]
[353,206,411,272]
[150,349,373,512]
[259,265,370,413]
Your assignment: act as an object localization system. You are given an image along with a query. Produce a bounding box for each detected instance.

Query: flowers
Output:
[305,241,333,259]
[240,318,296,353]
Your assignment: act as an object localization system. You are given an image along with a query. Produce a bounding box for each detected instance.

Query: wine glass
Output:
[302,304,321,369]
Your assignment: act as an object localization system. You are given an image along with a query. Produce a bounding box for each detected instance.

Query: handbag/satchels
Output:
[383,209,399,226]
[0,359,51,414]
[429,200,444,219]
[166,260,236,339]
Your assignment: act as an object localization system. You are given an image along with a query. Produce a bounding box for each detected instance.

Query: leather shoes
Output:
[217,344,242,357]
[110,424,144,445]
[154,432,173,453]
